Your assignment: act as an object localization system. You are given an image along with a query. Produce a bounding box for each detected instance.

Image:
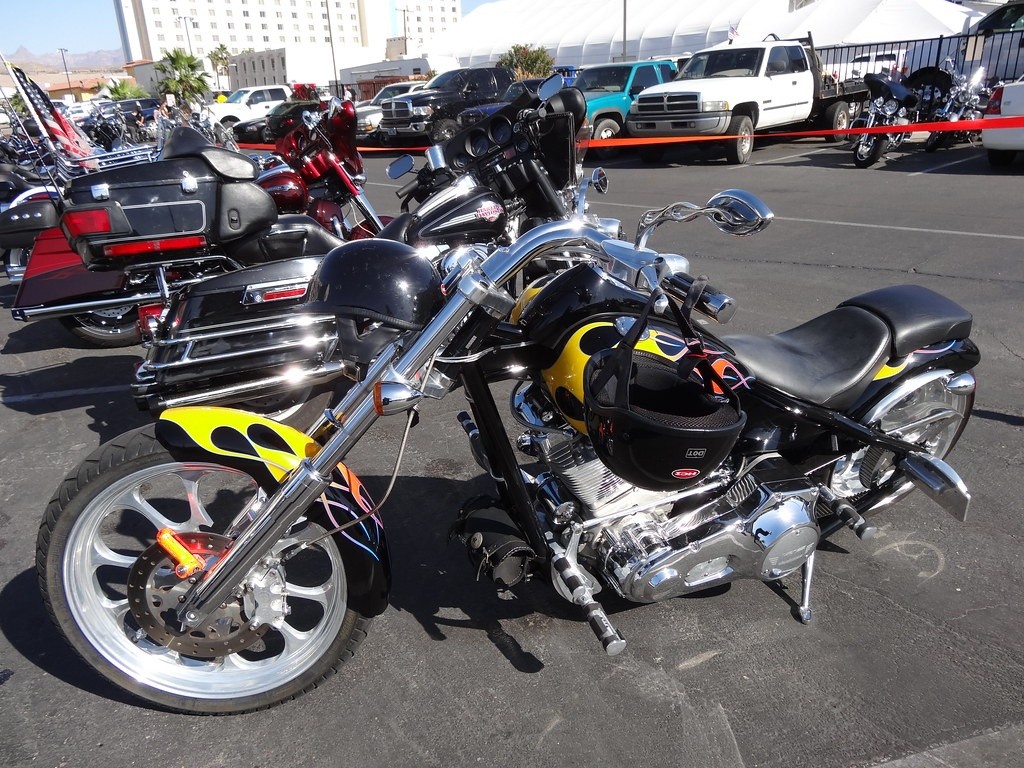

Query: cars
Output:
[232,116,275,144]
[264,100,325,138]
[981,73,1024,151]
[49,97,161,130]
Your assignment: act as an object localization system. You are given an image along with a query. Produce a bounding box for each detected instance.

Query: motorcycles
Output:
[924,64,988,150]
[29,179,986,718]
[1,48,398,346]
[845,69,920,169]
[53,74,625,420]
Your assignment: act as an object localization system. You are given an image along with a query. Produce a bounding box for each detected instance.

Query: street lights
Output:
[57,47,75,96]
[178,14,203,57]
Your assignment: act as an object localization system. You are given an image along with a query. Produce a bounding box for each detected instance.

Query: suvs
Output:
[378,67,518,148]
[355,80,429,145]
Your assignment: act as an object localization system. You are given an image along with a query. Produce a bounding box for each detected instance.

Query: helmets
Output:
[583,346,750,492]
[295,238,447,334]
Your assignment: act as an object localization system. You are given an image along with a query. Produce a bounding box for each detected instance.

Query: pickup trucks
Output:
[570,58,680,165]
[625,33,871,166]
[205,84,335,143]
[458,77,579,135]
